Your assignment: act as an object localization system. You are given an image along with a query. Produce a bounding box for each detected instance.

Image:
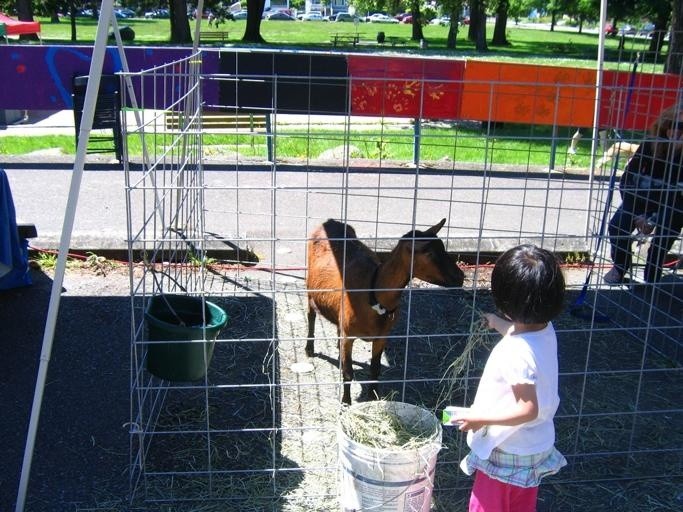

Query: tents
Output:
[0,21,9,44]
[0,12,43,45]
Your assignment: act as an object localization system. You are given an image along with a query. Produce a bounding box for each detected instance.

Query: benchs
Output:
[194,32,228,43]
[167,112,266,132]
[329,33,366,47]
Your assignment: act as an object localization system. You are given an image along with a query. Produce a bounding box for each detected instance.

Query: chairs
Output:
[72,74,123,160]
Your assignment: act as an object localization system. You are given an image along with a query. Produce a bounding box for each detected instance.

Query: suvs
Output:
[461,17,471,22]
[336,11,363,24]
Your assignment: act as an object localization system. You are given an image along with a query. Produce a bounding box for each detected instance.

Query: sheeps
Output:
[305,218,464,406]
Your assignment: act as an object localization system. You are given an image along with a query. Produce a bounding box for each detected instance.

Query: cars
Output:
[395,13,409,20]
[402,15,427,23]
[191,9,214,19]
[372,15,399,24]
[72,9,93,18]
[604,25,618,35]
[266,12,296,21]
[621,25,636,35]
[112,11,126,21]
[329,15,337,21]
[301,12,322,22]
[145,10,168,19]
[230,12,248,20]
[117,8,136,18]
[431,17,461,26]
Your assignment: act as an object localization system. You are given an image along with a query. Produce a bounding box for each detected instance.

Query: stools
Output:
[381,36,408,49]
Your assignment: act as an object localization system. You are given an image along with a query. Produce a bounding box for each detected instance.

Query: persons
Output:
[450,243,569,512]
[602,101,682,286]
[566,128,612,156]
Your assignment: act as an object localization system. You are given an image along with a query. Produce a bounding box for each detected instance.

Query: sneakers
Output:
[603,266,627,286]
[567,147,576,154]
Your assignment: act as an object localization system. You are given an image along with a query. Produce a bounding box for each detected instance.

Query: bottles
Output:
[637,213,659,242]
[442,407,473,427]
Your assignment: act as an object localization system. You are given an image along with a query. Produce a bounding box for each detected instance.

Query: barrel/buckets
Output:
[338,400,443,511]
[144,268,227,382]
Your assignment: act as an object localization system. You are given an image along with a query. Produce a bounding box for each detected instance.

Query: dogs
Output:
[595,142,639,170]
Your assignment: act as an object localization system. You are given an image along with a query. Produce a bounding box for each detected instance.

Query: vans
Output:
[638,23,656,36]
